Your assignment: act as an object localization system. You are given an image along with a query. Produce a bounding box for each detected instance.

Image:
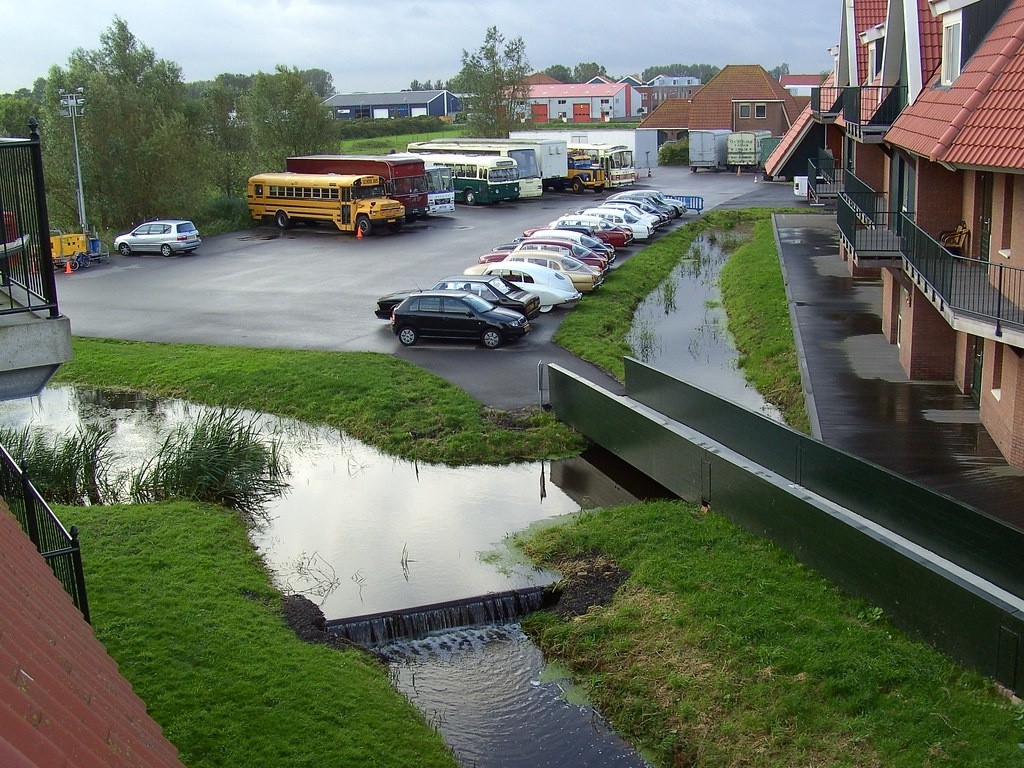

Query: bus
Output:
[569,142,636,189]
[248,173,405,235]
[387,152,520,206]
[286,154,432,223]
[431,138,568,191]
[407,141,543,201]
[417,165,455,218]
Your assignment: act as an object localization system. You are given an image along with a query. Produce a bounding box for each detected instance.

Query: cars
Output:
[463,249,605,292]
[391,290,529,349]
[114,219,201,257]
[478,238,608,272]
[374,274,541,321]
[491,189,687,264]
[481,261,582,313]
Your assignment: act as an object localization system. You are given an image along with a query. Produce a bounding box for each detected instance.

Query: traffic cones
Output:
[648,168,651,177]
[736,166,741,176]
[65,262,73,274]
[637,173,640,179]
[356,226,363,238]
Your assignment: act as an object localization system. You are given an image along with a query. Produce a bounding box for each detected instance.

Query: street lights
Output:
[58,87,85,233]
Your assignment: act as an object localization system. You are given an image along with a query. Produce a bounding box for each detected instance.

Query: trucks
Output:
[564,149,605,194]
[687,130,732,173]
[51,233,87,267]
[760,137,817,182]
[509,129,659,170]
[727,130,773,173]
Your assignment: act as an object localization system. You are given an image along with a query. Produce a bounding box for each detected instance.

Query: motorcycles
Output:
[69,251,91,271]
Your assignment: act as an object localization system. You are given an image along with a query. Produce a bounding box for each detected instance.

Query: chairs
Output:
[938,228,970,263]
[934,220,967,249]
